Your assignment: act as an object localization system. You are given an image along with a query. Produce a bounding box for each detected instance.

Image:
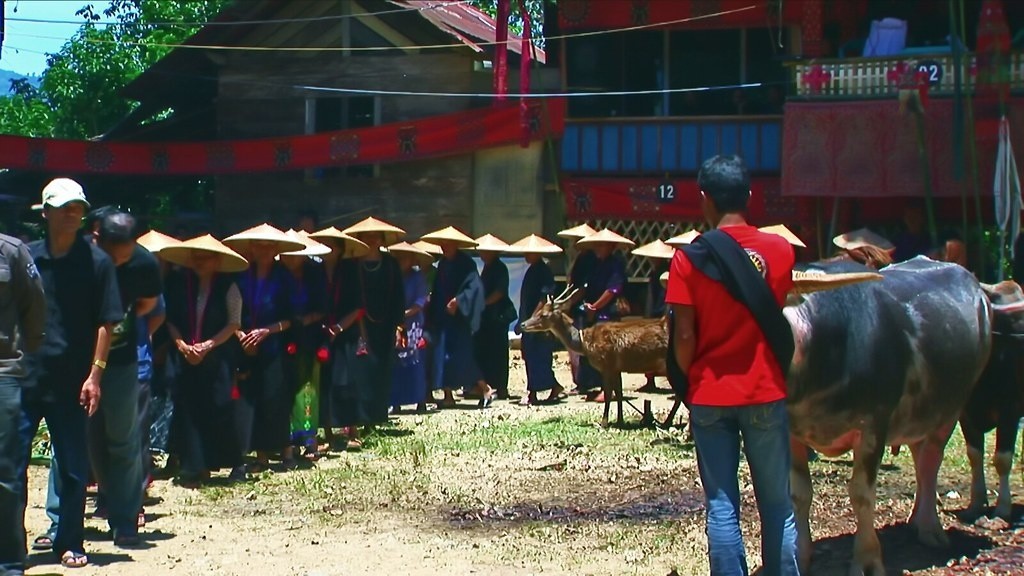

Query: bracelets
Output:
[337,324,344,332]
[92,359,106,369]
[328,328,336,337]
[454,298,456,301]
[279,321,282,331]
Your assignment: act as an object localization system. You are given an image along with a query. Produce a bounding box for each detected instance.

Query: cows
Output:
[777,261,991,576]
[960,281,1024,517]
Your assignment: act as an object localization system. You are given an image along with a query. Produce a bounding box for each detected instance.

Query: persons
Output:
[0,178,671,576]
[848,199,929,268]
[664,149,802,576]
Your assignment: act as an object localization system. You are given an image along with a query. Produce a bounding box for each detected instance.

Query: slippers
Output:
[31,531,57,548]
[57,548,88,568]
[94,387,569,546]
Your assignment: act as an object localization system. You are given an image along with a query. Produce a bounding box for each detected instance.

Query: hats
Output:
[557,223,597,239]
[420,226,479,248]
[757,222,807,248]
[664,229,702,245]
[630,238,677,259]
[41,177,91,210]
[465,233,564,255]
[159,233,251,273]
[575,227,636,249]
[222,216,445,266]
[136,229,182,252]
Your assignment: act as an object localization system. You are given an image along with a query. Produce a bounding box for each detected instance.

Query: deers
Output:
[519,285,681,428]
